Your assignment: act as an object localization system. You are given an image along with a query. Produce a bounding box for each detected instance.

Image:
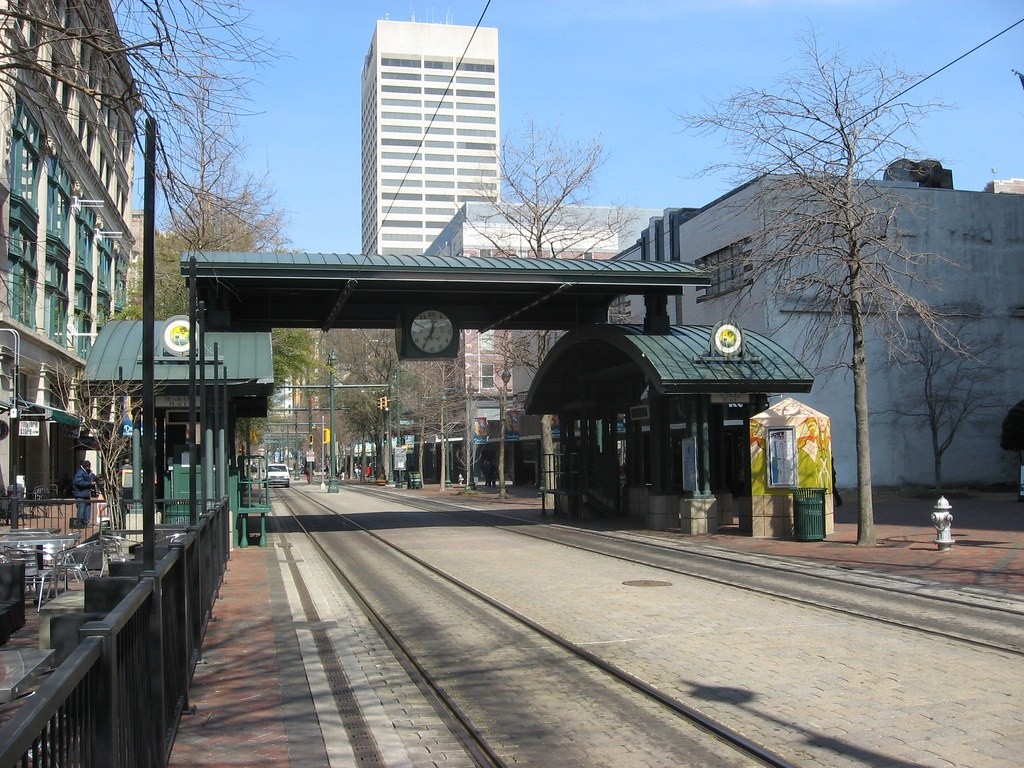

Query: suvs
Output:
[261,464,291,489]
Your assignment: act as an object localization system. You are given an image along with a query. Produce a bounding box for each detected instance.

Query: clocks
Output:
[409,308,454,355]
[394,314,404,354]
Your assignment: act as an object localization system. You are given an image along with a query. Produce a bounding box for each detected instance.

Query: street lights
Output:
[440,394,455,488]
[386,397,396,485]
[497,367,510,493]
[466,382,478,491]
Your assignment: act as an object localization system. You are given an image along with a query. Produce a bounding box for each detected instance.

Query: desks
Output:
[0,530,81,604]
[0,528,62,569]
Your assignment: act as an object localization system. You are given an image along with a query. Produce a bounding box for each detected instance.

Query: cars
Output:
[313,467,328,476]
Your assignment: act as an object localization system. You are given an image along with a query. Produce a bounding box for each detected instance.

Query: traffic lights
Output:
[377,397,382,409]
[382,396,388,409]
[309,435,314,447]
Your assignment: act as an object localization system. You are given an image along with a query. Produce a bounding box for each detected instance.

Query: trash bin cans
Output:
[790,487,829,543]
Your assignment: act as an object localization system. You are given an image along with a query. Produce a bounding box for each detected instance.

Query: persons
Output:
[324,462,372,485]
[831,452,842,506]
[304,457,314,484]
[72,460,97,526]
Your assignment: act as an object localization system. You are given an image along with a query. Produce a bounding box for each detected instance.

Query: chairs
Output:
[1,534,145,613]
[0,562,25,647]
[0,483,61,527]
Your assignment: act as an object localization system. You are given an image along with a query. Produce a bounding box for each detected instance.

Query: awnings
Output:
[10,397,82,426]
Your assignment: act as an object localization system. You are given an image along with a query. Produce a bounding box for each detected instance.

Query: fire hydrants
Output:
[930,496,957,553]
